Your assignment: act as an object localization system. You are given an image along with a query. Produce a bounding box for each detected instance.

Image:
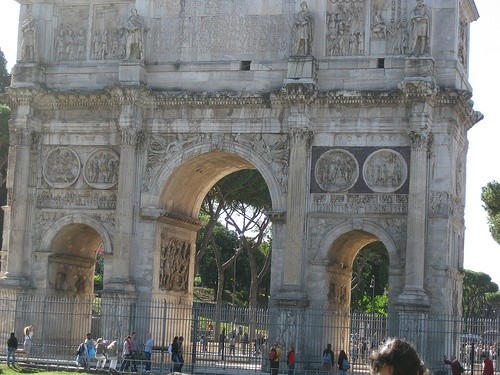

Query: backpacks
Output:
[269,346,277,360]
[459,362,464,374]
[321,352,332,370]
[342,356,350,371]
[167,344,173,354]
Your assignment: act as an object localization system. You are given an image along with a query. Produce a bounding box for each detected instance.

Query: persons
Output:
[350,332,385,361]
[323,344,334,375]
[106,340,119,373]
[22,325,33,362]
[7,332,18,367]
[461,330,497,363]
[369,337,430,375]
[143,332,154,372]
[177,336,185,373]
[196,323,266,359]
[444,355,465,375]
[482,354,494,375]
[286,345,296,375]
[124,331,142,372]
[337,350,348,375]
[268,342,283,375]
[95,338,107,371]
[120,335,135,372]
[171,336,179,373]
[83,333,95,360]
